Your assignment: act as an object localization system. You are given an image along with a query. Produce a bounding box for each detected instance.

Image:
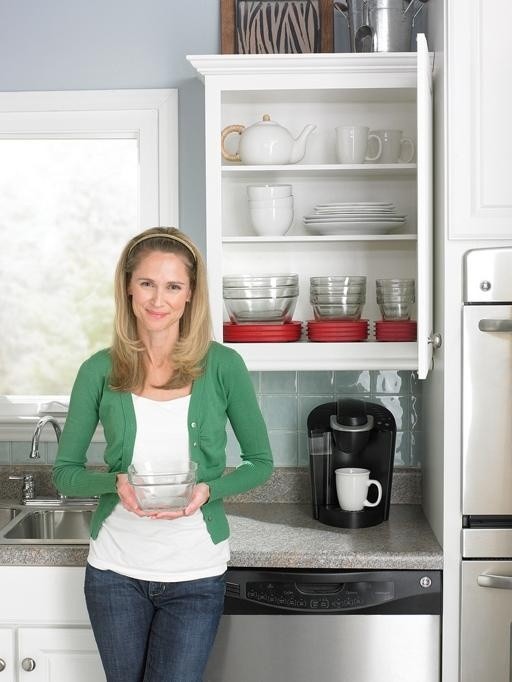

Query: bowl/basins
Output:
[222,273,301,324]
[126,458,199,514]
[308,275,367,320]
[375,277,416,321]
[245,183,295,237]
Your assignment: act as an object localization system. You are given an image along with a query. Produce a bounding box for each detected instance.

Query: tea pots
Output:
[220,113,317,166]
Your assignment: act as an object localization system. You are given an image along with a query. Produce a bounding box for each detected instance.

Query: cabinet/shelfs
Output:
[0,565,108,682]
[426,2,512,242]
[186,33,435,380]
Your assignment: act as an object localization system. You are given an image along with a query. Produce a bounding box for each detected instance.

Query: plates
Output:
[302,202,408,235]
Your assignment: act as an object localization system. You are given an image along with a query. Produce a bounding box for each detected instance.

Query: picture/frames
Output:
[221,1,334,55]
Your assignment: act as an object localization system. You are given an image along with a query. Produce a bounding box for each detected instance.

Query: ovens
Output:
[203,569,443,682]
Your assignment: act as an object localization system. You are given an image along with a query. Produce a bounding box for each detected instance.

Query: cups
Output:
[369,128,415,163]
[334,125,383,164]
[334,466,384,512]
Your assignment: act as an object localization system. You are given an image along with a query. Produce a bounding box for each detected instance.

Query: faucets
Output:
[29,415,65,500]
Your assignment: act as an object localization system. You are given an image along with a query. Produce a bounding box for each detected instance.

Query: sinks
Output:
[0,501,25,531]
[2,505,101,541]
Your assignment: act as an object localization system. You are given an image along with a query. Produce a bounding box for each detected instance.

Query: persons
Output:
[50,225,273,680]
